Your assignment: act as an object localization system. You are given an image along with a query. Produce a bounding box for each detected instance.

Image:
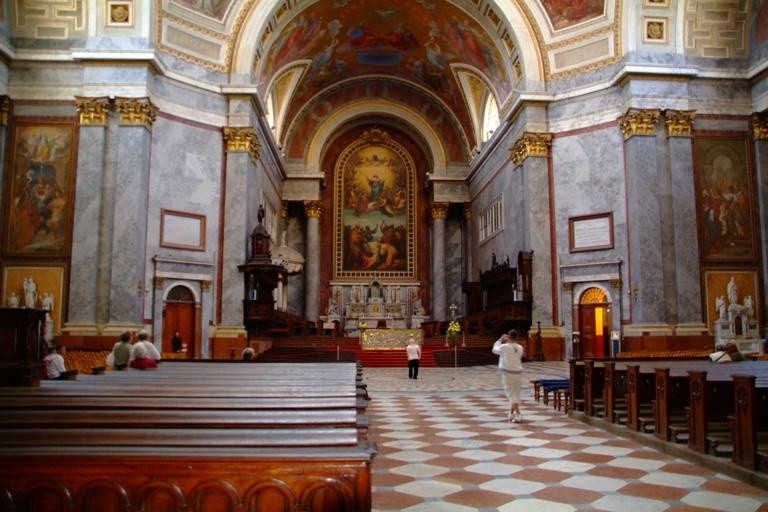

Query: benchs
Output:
[530,370,768,473]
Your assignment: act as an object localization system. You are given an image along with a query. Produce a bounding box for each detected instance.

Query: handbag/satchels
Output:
[129,358,158,370]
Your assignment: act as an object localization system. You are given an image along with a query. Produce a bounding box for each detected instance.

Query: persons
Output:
[345,154,406,271]
[130,345,157,369]
[39,292,53,320]
[23,276,36,309]
[492,329,523,423]
[702,179,751,252]
[47,345,78,380]
[8,291,20,308]
[173,331,181,351]
[113,331,132,370]
[131,329,161,360]
[727,276,739,304]
[12,166,41,247]
[44,190,66,231]
[716,296,726,319]
[407,338,422,379]
[243,347,255,361]
[42,347,56,378]
[744,295,754,316]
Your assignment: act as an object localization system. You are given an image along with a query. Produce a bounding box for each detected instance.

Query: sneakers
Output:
[507,412,524,423]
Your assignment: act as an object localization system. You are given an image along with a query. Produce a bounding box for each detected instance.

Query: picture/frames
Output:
[568,211,614,252]
[161,208,206,251]
